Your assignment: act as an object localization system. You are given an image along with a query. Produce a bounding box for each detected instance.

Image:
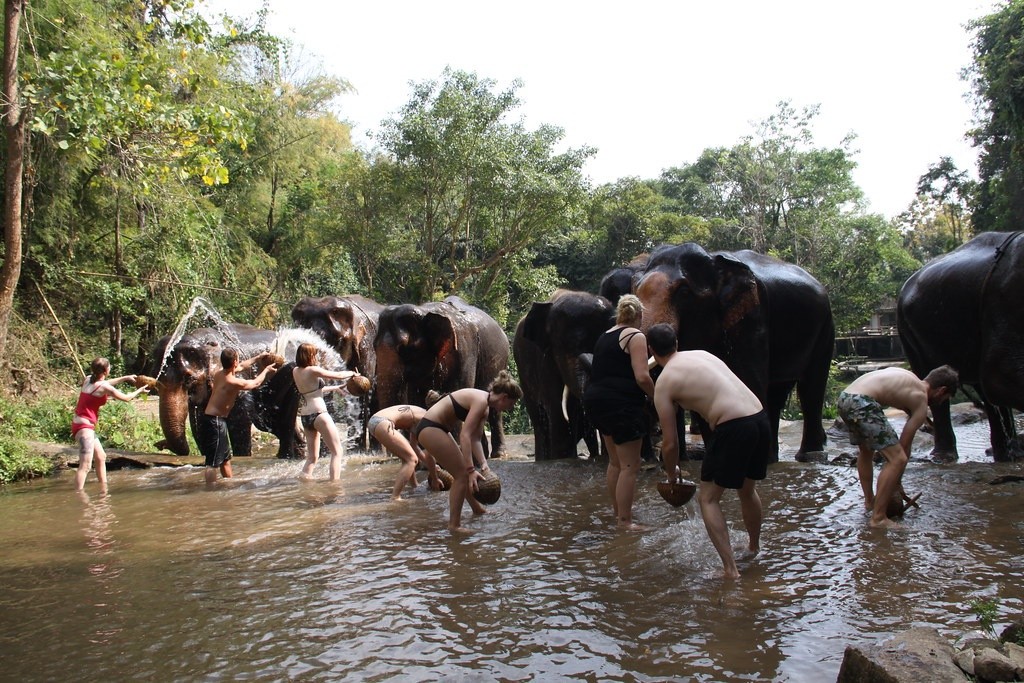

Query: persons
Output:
[646,323,771,581]
[292,343,361,481]
[199,348,278,485]
[588,292,657,529]
[71,359,150,494]
[835,364,959,534]
[367,389,445,502]
[415,371,524,530]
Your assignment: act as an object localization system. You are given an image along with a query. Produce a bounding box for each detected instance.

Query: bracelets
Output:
[467,466,477,474]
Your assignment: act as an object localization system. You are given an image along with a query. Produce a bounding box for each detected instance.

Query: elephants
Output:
[514,243,837,461]
[145,293,510,459]
[895,230,1024,463]
